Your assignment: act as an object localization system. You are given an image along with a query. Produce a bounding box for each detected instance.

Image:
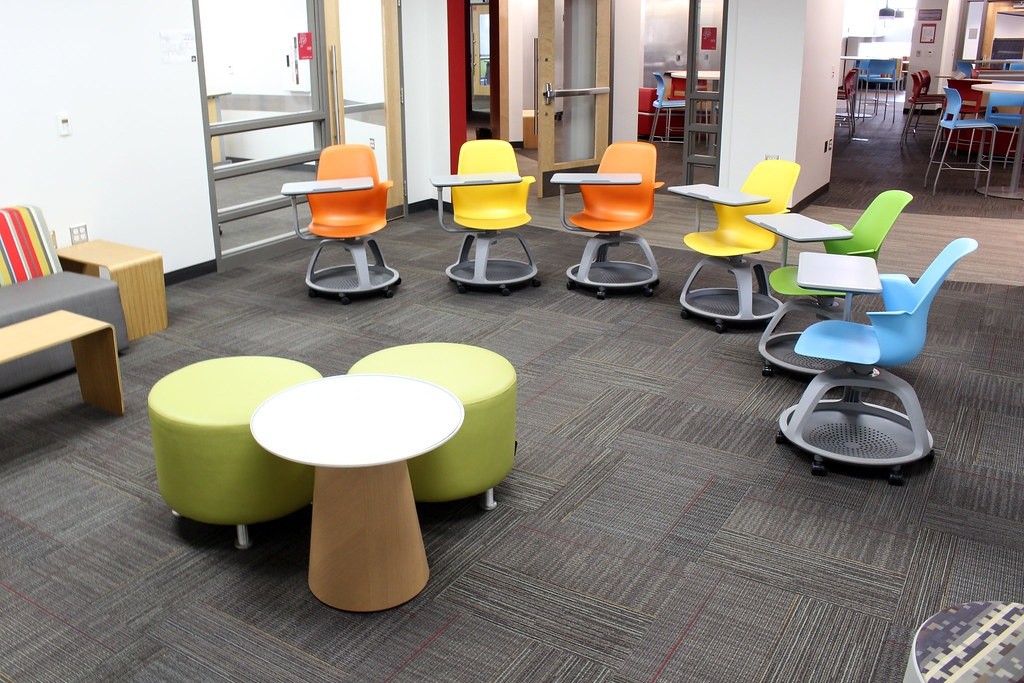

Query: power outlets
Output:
[69,225,89,246]
[369,138,375,149]
[765,154,779,160]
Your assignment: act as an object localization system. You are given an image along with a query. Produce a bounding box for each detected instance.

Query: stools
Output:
[147,356,325,550]
[346,342,517,512]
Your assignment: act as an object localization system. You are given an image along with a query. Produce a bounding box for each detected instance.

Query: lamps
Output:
[878,0,895,19]
[895,9,904,18]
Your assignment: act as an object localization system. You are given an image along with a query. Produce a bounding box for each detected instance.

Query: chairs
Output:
[566,141,660,299]
[649,72,708,149]
[837,59,897,143]
[758,190,913,378]
[445,139,541,297]
[900,60,1024,197]
[306,144,401,305]
[774,237,979,485]
[680,159,800,335]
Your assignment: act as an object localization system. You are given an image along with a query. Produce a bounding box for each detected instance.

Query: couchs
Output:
[0,205,131,398]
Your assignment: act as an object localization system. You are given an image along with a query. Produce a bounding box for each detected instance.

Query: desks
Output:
[550,171,642,235]
[671,71,721,169]
[796,251,883,322]
[57,239,169,340]
[934,59,1024,200]
[0,309,126,415]
[279,177,373,239]
[668,183,770,232]
[249,374,464,612]
[429,173,522,233]
[840,57,891,120]
[745,213,855,267]
